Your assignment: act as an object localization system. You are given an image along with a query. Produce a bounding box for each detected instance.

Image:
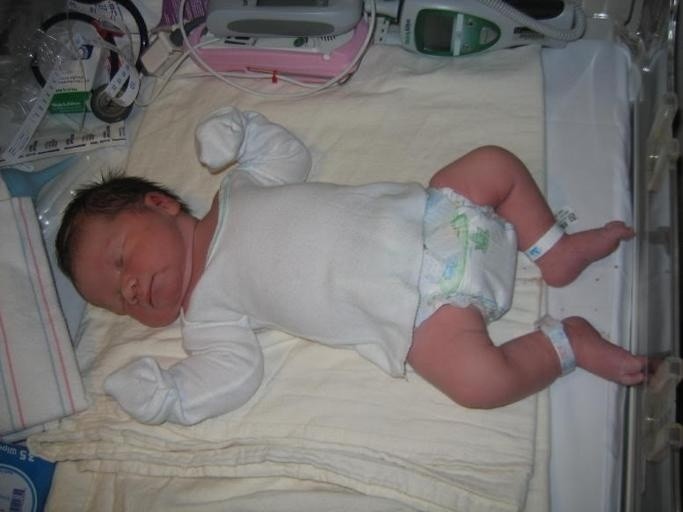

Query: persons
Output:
[54,108,651,425]
